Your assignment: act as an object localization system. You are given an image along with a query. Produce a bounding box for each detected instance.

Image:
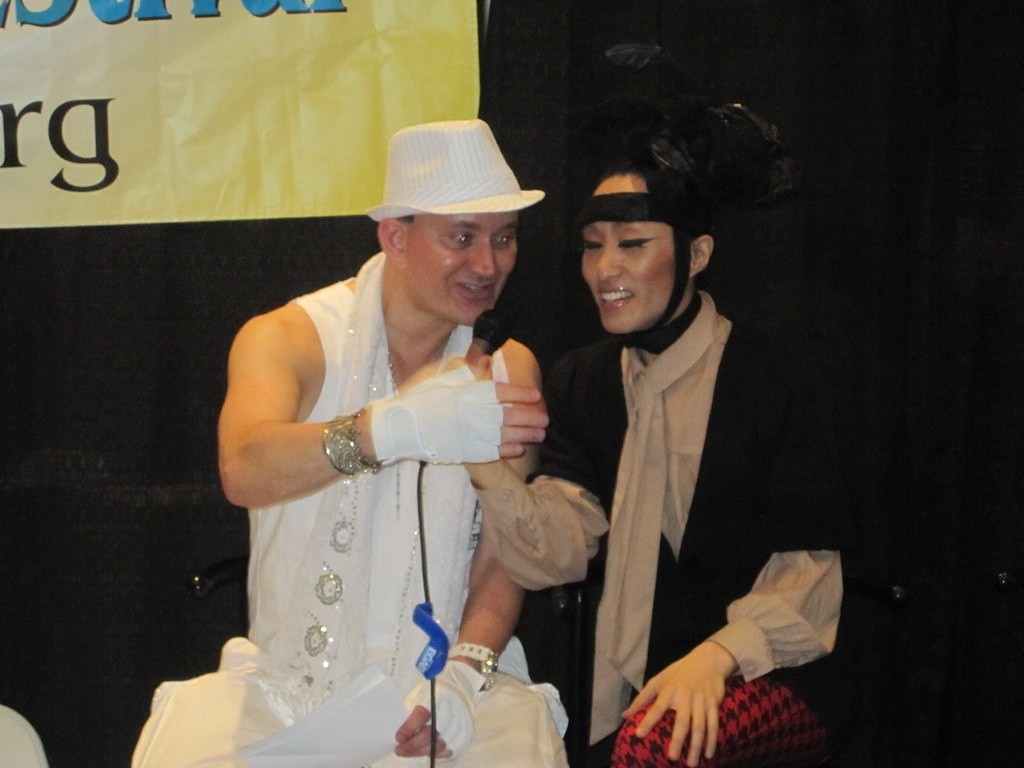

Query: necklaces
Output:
[387,351,398,389]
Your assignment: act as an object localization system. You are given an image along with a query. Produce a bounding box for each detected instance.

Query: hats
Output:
[367,120,546,221]
[581,44,796,239]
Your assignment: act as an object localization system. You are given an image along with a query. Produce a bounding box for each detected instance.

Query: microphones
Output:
[464,309,512,366]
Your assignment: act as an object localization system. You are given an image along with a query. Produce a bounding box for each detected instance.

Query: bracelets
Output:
[322,412,380,479]
[448,642,498,690]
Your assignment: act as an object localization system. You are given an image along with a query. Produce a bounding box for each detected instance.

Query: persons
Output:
[462,89,851,768]
[128,117,571,766]
